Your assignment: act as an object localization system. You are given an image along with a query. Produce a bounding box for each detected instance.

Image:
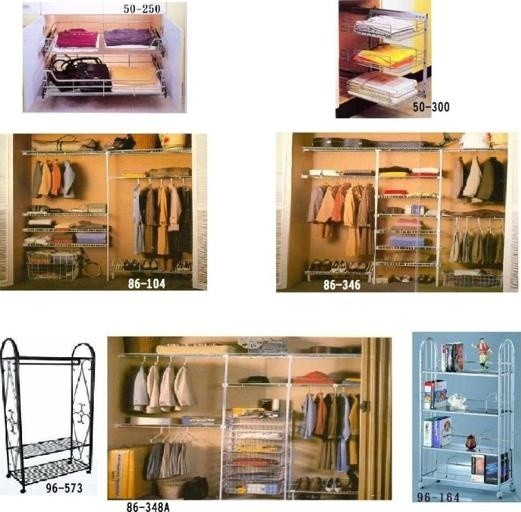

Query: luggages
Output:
[108,446,154,499]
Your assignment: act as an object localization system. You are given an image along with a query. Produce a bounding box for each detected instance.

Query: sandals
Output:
[419,274,433,283]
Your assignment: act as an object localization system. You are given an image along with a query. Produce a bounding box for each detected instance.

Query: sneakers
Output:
[391,275,410,282]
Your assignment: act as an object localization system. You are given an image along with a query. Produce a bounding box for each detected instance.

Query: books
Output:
[424,417,451,448]
[472,453,509,485]
[425,379,448,409]
[440,342,463,371]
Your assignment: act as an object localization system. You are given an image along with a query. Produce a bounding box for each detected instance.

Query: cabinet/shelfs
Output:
[19,144,192,283]
[345,7,429,111]
[0,336,96,487]
[40,22,169,101]
[418,336,515,489]
[300,143,507,288]
[113,349,362,500]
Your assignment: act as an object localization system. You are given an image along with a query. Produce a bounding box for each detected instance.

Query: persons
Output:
[472,338,492,372]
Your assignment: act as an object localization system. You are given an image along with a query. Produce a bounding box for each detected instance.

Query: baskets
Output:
[156,480,185,499]
[132,134,158,150]
[444,273,498,290]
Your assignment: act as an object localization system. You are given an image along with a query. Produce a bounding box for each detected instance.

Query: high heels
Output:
[293,468,358,500]
[124,257,191,272]
[108,135,135,150]
[310,258,367,273]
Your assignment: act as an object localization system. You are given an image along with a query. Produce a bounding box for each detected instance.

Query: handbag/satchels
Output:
[50,54,112,92]
[32,136,99,152]
[182,476,208,500]
